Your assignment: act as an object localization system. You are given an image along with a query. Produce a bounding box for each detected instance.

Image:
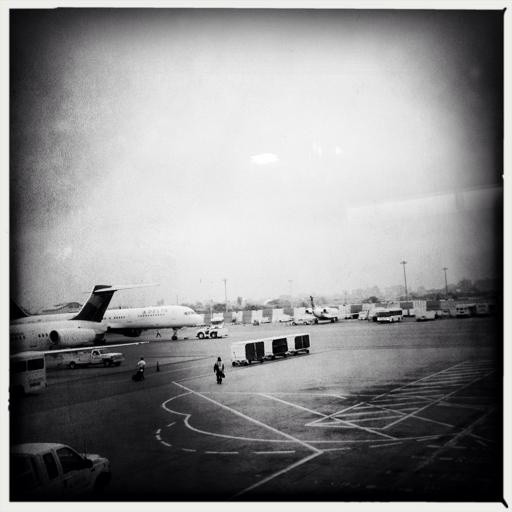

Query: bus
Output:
[376,308,403,323]
[10,350,48,396]
[416,304,495,321]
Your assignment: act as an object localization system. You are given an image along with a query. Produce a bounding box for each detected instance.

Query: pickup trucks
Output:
[10,440,113,501]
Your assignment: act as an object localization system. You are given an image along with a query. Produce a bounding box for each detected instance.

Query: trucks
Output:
[64,348,125,370]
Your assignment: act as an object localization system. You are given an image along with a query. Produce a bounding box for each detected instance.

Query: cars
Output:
[291,317,310,326]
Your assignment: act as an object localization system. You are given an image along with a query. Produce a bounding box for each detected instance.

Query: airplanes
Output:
[304,295,369,326]
[10,282,151,359]
[10,296,203,340]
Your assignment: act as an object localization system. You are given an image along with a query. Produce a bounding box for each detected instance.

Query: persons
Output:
[155,329,162,338]
[214,357,224,384]
[136,357,146,375]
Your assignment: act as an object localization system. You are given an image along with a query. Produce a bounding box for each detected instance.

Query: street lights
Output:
[442,266,448,299]
[400,260,410,318]
